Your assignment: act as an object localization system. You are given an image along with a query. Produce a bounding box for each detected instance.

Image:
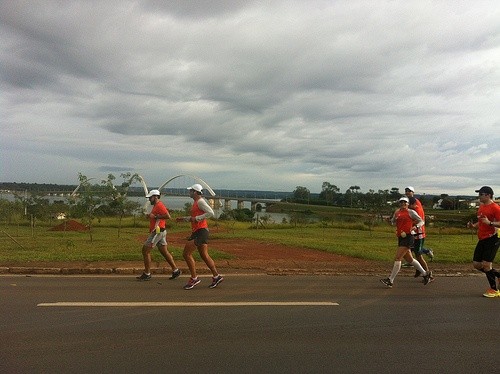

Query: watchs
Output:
[490,221,494,226]
[154,215,158,219]
[415,224,420,230]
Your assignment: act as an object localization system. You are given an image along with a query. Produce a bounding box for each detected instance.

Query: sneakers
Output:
[482,288,500,297]
[426,249,435,262]
[422,272,431,286]
[414,266,425,278]
[428,271,433,283]
[183,277,201,290]
[208,275,224,289]
[136,272,151,280]
[401,262,415,268]
[380,277,393,288]
[495,270,500,284]
[169,269,181,280]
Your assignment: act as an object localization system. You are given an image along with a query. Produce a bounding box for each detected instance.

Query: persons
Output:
[136,189,182,281]
[380,186,434,288]
[176,184,223,290]
[467,186,500,297]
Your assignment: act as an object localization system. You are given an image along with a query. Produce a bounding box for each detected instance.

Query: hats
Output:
[145,190,160,198]
[399,197,409,203]
[405,186,415,193]
[475,186,493,195]
[187,184,204,195]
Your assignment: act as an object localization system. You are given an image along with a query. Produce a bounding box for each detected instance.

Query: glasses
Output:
[405,190,412,192]
[479,193,488,196]
